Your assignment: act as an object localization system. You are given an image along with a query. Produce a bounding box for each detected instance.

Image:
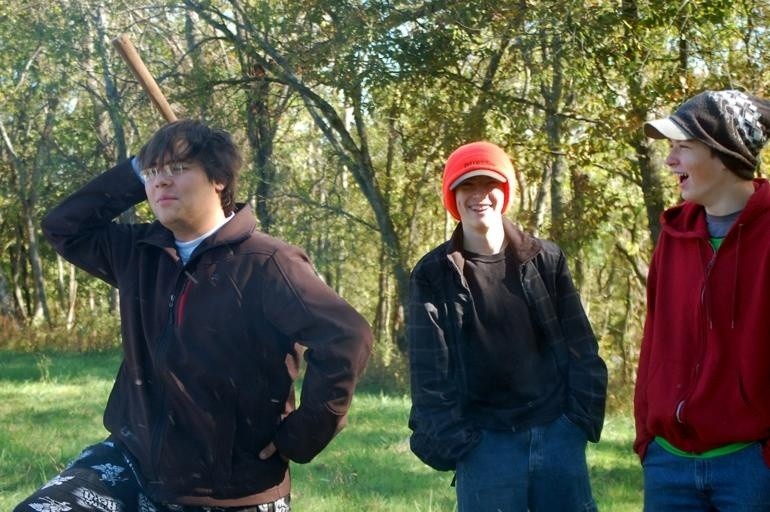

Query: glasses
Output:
[140,163,191,182]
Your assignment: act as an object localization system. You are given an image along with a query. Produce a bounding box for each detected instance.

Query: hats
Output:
[644,91,770,180]
[443,143,514,220]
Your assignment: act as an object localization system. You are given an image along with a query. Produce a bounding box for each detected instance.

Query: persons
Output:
[631,87,768,511]
[11,120,374,512]
[399,138,608,512]
